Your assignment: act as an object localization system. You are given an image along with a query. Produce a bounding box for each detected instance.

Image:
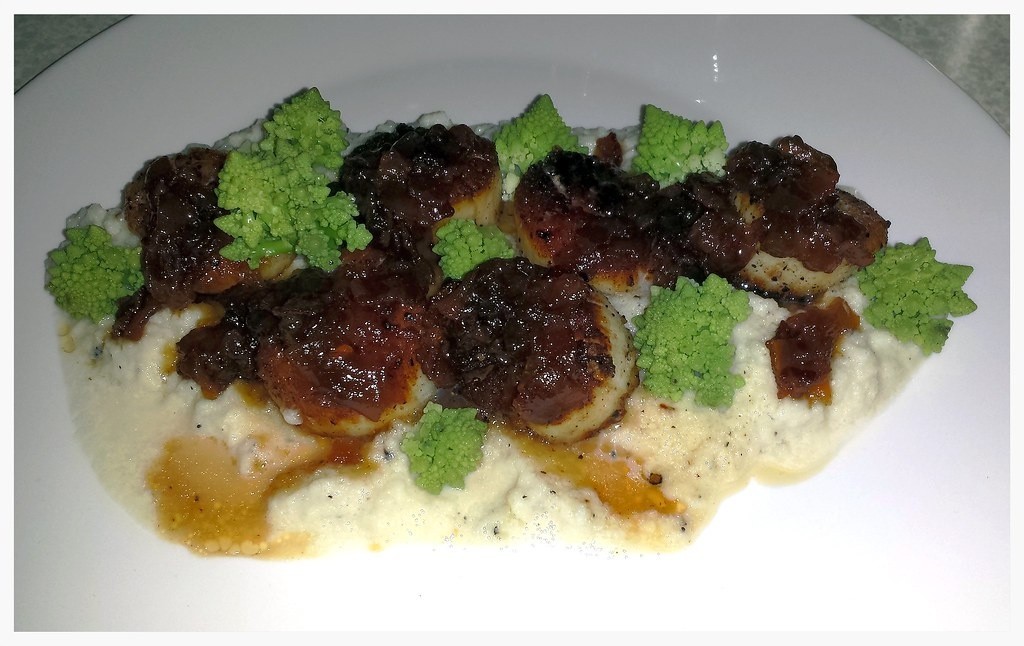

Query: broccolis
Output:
[38,86,976,497]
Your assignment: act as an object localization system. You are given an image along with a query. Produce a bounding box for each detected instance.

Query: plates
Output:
[14,14,1008,631]
